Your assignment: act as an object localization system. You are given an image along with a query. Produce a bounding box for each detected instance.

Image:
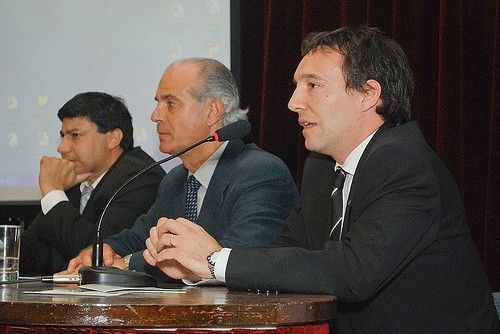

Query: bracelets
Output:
[123,254,131,270]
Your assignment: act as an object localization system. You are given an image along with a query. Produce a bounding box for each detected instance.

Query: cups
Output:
[0,224,22,284]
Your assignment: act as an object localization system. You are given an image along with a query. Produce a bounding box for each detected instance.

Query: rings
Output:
[170,235,176,248]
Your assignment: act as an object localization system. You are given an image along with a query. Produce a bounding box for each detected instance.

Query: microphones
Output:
[78,119,252,286]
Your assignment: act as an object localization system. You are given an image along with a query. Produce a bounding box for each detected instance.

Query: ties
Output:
[185,174,202,224]
[330,166,348,243]
[79,185,94,215]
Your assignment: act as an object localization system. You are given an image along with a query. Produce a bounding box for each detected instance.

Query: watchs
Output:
[207,248,222,280]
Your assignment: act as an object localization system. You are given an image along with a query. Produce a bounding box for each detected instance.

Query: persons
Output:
[143,24,500,334]
[52,57,300,287]
[7,92,167,277]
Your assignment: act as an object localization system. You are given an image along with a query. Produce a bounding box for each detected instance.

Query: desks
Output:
[0,275,337,334]
